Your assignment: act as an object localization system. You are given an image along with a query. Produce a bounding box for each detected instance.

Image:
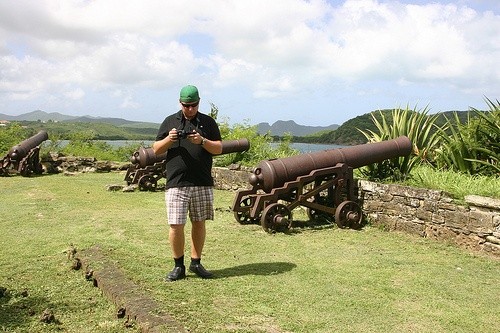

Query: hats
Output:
[180,85,199,102]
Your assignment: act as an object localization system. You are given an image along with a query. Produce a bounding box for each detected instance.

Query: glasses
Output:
[181,101,199,108]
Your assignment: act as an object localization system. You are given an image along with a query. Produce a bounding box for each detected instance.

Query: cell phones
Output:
[179,131,196,139]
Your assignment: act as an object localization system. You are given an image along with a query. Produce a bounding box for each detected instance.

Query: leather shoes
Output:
[168,265,185,280]
[189,264,214,277]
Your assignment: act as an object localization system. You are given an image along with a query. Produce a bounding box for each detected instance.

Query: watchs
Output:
[199,137,206,146]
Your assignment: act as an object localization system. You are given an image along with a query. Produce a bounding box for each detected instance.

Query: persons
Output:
[152,83,223,282]
